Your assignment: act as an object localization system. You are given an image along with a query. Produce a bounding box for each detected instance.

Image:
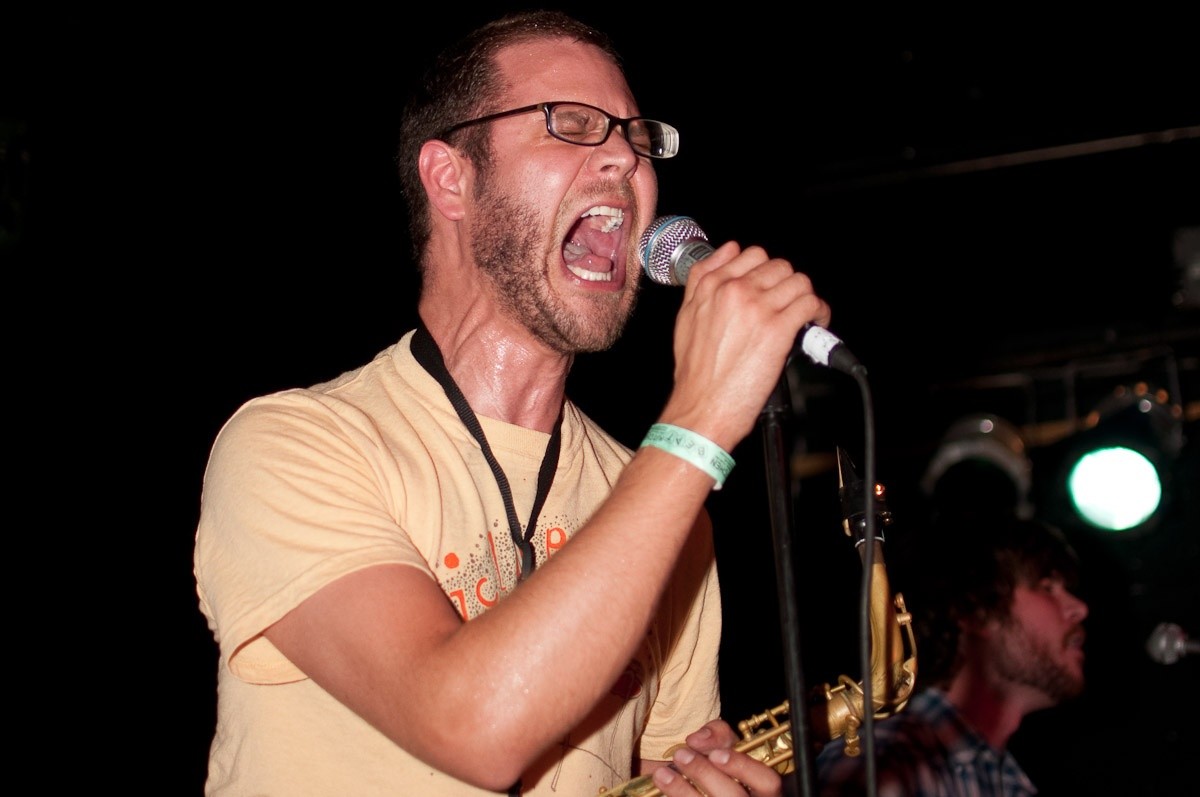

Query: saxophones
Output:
[594,443,918,797]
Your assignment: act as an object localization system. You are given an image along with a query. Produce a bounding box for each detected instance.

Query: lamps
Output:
[1066,398,1176,533]
[922,416,1031,524]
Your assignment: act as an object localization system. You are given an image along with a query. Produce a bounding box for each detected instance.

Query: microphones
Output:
[639,214,868,379]
[1147,624,1200,666]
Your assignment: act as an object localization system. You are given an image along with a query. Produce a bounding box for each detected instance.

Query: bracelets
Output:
[640,423,737,491]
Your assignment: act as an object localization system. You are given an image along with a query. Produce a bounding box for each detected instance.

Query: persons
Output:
[192,13,831,797]
[817,520,1087,797]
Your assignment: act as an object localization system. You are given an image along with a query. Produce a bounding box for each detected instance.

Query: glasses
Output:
[436,101,680,160]
